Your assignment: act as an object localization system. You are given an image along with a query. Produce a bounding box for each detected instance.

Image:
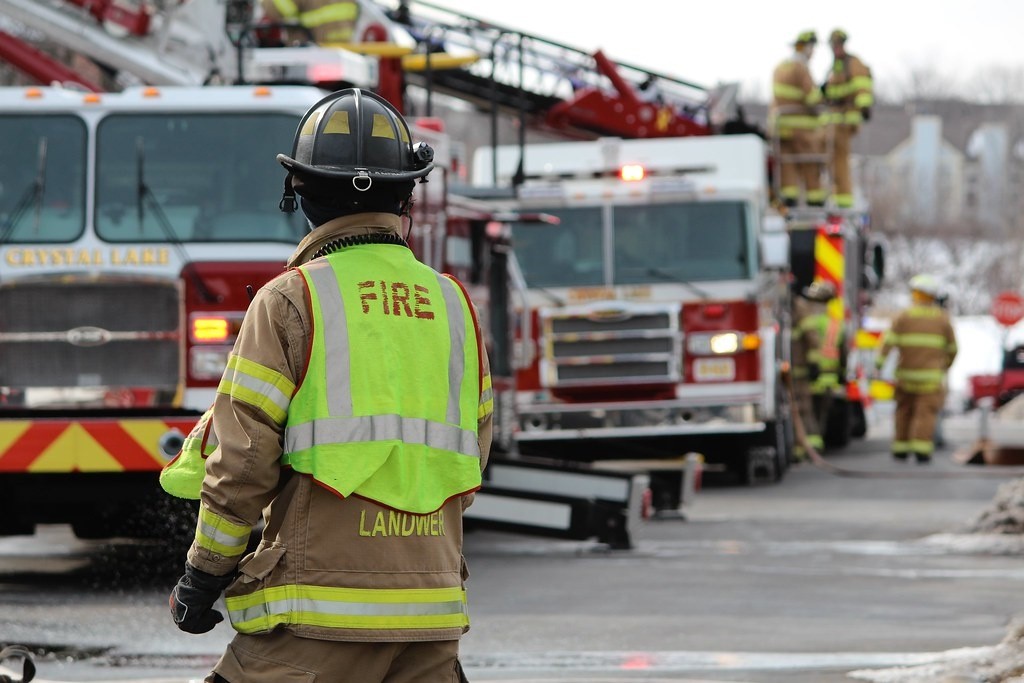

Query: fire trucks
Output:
[0,2,886,555]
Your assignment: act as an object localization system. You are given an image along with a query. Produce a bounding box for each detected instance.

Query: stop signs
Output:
[993,293,1024,325]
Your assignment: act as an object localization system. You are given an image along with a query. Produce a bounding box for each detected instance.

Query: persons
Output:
[771,30,827,207]
[821,27,876,206]
[166,87,496,683]
[875,271,958,465]
[792,274,849,400]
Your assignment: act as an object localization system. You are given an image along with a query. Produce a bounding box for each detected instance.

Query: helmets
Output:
[275,88,436,192]
[799,280,837,304]
[908,272,939,301]
[792,30,818,46]
[830,28,848,42]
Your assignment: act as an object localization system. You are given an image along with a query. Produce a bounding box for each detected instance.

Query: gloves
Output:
[860,106,870,119]
[169,561,240,635]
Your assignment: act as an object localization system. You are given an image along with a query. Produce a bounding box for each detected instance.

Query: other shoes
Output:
[913,451,930,463]
[893,450,909,459]
